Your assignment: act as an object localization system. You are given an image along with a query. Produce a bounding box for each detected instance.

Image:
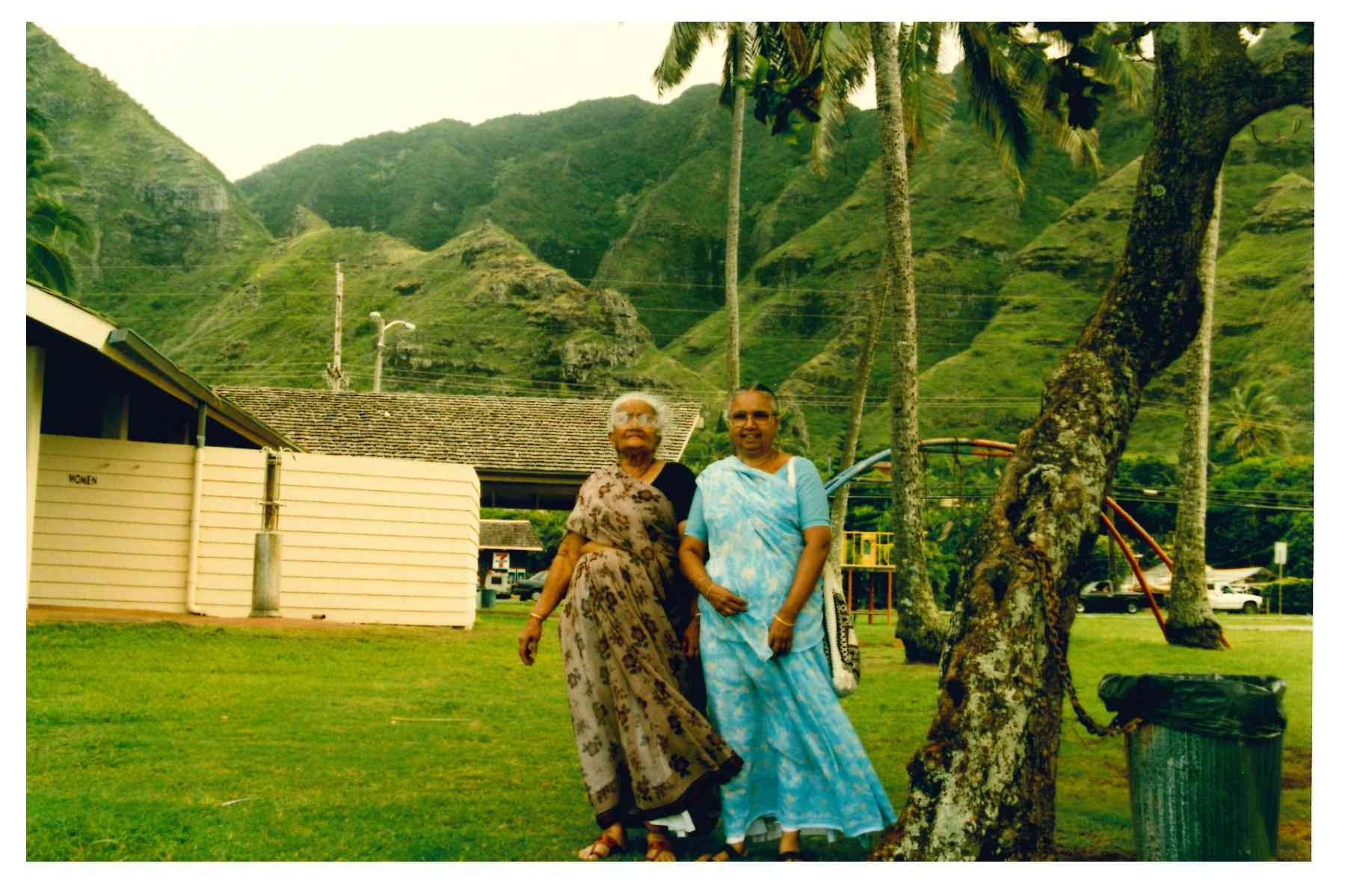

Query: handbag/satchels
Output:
[820,560,861,698]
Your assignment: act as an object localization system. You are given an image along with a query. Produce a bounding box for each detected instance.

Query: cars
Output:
[511,569,550,601]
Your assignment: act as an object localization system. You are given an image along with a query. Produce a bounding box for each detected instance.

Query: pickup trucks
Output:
[1075,580,1165,614]
[1206,582,1264,615]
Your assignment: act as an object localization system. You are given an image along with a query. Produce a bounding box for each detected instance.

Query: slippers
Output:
[578,834,622,861]
[695,843,748,861]
[645,842,677,861]
[778,850,804,861]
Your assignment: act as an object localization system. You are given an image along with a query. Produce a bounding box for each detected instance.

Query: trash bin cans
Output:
[1098,674,1286,862]
[481,590,496,608]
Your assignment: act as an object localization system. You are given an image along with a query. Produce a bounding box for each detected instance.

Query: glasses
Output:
[728,411,779,429]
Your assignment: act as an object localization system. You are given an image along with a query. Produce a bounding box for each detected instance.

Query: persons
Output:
[678,382,898,862]
[518,390,745,862]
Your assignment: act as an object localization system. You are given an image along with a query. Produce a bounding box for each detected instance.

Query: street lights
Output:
[369,311,417,393]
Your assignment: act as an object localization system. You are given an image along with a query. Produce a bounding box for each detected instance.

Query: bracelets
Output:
[773,615,795,627]
[704,586,715,598]
[530,613,544,620]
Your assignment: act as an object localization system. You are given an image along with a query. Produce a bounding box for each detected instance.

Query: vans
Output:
[485,573,511,598]
